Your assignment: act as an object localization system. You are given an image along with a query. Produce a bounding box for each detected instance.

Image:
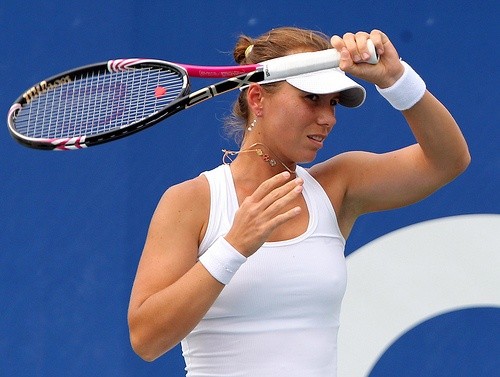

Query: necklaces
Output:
[221,142,297,174]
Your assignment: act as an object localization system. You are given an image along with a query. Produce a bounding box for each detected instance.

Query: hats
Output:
[239,66,366,109]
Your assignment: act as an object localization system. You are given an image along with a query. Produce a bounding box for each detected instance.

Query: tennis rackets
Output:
[7,38,381,151]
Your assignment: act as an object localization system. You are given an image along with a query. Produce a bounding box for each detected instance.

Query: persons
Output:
[127,27,472,377]
